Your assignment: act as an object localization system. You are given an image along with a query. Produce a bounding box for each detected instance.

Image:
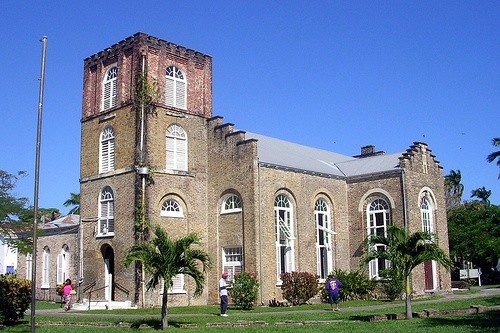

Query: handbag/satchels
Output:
[70,288,76,295]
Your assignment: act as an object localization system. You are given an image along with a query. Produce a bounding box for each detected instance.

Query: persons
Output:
[326,275,342,312]
[63,279,74,311]
[219,272,233,317]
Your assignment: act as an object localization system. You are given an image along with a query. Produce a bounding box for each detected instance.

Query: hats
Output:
[222,274,228,277]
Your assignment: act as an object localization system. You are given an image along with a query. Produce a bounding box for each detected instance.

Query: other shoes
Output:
[220,314,229,317]
